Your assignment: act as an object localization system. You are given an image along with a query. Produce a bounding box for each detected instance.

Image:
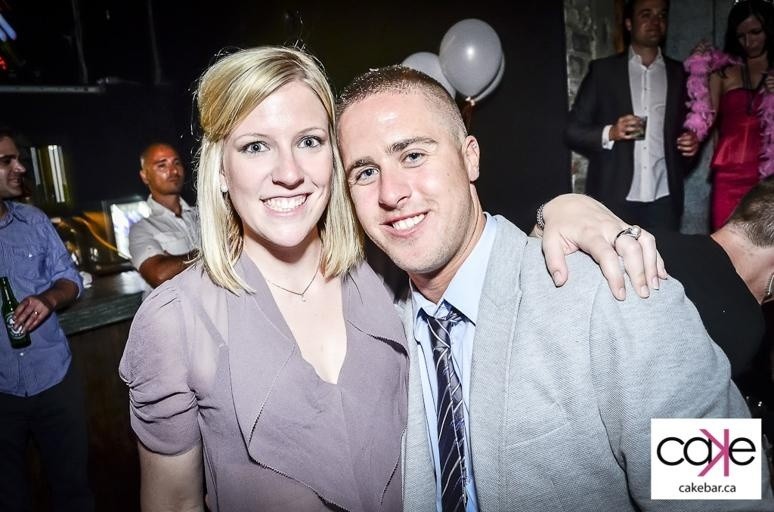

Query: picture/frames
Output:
[101,195,144,265]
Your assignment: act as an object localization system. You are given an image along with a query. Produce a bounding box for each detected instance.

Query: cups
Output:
[632,116,647,140]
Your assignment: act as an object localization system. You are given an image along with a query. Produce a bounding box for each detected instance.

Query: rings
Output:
[32,311,40,315]
[613,225,641,257]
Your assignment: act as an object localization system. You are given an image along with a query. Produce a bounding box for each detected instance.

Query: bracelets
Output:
[534,224,542,239]
[536,203,545,230]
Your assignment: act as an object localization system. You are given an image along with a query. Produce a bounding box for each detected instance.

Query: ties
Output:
[418,305,468,512]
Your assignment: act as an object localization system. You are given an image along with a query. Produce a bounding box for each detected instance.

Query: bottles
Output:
[0,277,31,349]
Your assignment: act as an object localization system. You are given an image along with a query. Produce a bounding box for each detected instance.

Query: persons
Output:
[116,41,676,511]
[128,141,202,303]
[680,0,774,232]
[564,2,703,231]
[334,63,773,512]
[0,131,98,512]
[643,176,772,382]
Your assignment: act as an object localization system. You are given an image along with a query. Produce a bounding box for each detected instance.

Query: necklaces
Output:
[267,251,326,303]
